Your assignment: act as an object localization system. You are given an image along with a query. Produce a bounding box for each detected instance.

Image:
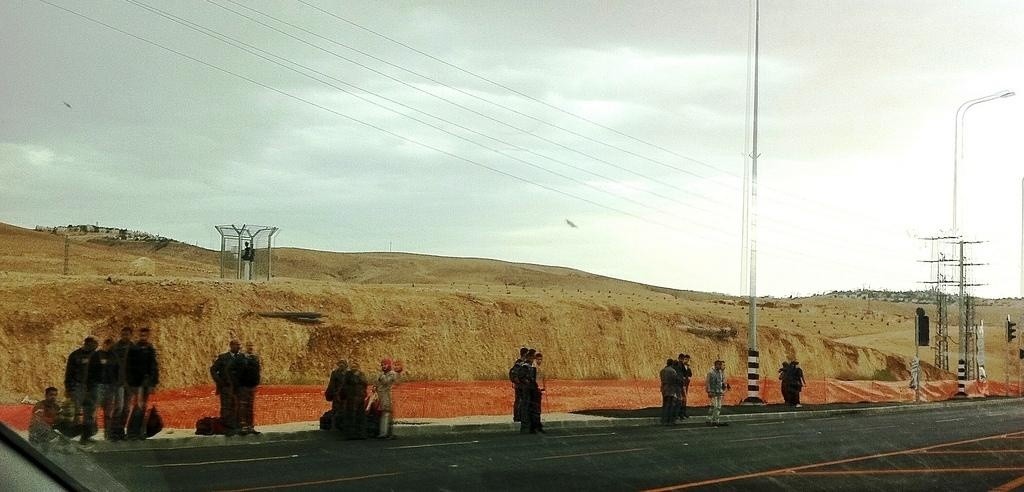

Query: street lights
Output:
[950,90,1016,296]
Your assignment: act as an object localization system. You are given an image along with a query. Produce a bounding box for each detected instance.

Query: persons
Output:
[507,345,552,436]
[322,356,411,439]
[651,352,733,428]
[208,339,246,435]
[224,340,261,436]
[28,327,160,445]
[239,241,252,280]
[772,358,809,412]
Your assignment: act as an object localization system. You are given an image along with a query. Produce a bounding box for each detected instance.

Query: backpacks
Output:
[146,405,164,438]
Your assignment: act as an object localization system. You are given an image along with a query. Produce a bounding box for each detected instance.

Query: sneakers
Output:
[42,430,128,452]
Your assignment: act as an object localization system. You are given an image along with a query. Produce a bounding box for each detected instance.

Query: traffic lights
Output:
[918,316,929,345]
[1019,348,1024,358]
[1008,322,1017,341]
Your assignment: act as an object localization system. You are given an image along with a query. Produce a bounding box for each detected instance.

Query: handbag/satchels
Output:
[195,416,227,436]
